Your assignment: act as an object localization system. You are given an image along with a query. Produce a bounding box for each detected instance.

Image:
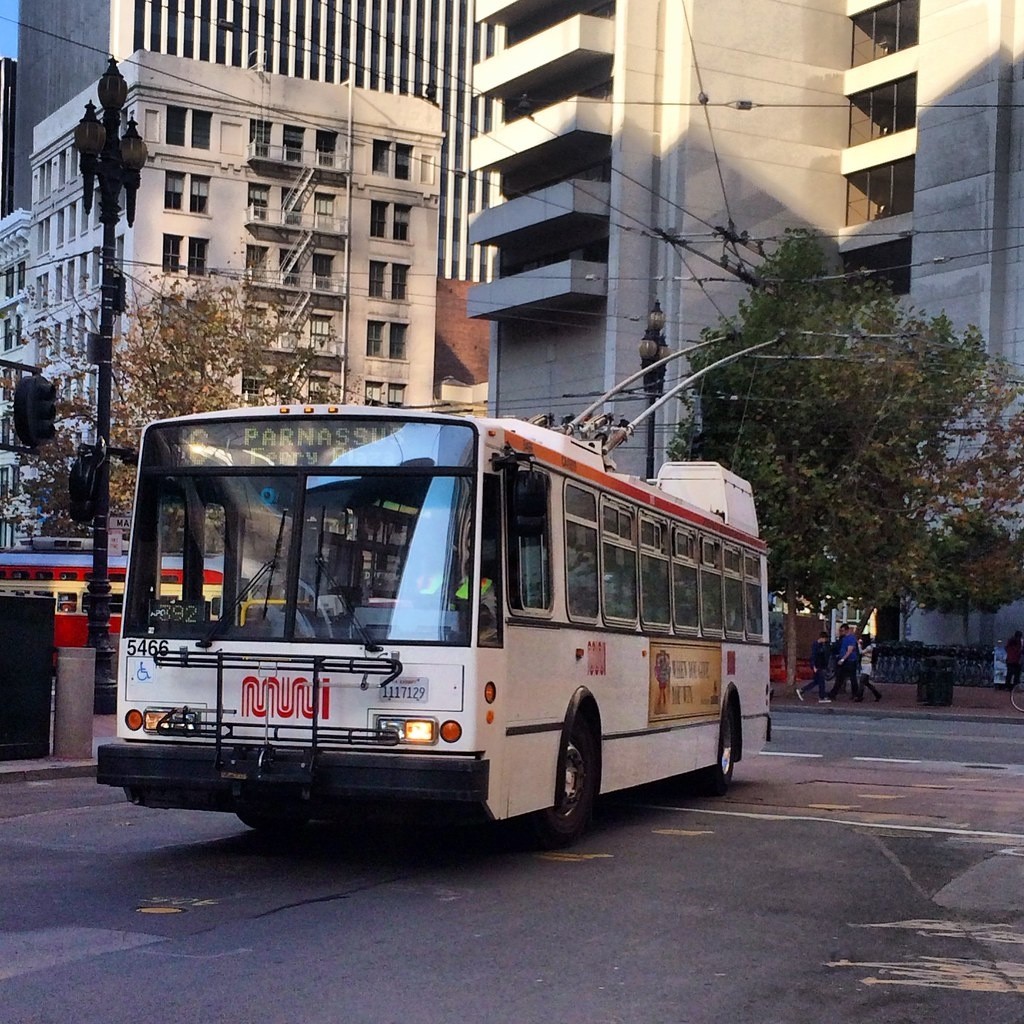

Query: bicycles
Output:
[824,639,1024,712]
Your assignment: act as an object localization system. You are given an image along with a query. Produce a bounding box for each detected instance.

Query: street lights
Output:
[74,53,148,714]
[640,299,671,479]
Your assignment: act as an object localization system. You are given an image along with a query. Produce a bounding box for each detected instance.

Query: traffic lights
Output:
[14,374,57,446]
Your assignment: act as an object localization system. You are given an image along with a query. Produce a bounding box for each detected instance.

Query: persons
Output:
[414,536,497,644]
[1003,631,1024,690]
[855,635,882,703]
[826,623,859,701]
[796,631,831,704]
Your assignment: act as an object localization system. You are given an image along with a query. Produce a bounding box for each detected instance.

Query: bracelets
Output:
[841,659,844,662]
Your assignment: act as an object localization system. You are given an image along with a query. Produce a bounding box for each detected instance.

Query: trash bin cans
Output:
[0,590,56,760]
[917,655,953,707]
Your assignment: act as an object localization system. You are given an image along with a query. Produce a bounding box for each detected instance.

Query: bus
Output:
[97,404,772,848]
[0,537,334,677]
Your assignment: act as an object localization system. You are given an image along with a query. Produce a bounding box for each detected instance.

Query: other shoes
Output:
[875,695,882,703]
[818,697,831,704]
[854,697,863,702]
[795,688,805,702]
